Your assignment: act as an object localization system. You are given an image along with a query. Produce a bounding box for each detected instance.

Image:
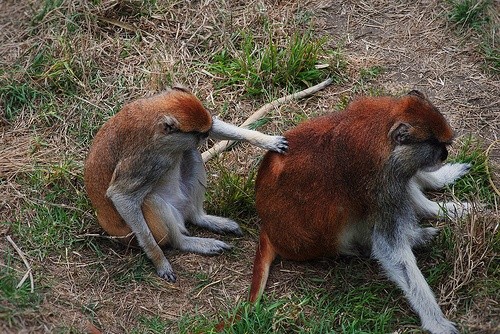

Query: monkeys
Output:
[213,87,495,334]
[86,86,290,285]
